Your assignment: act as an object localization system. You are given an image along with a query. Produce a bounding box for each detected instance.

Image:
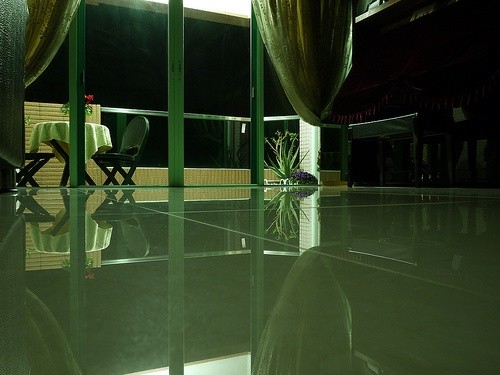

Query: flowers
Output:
[261,186,318,240]
[61,94,93,119]
[61,258,96,281]
[262,129,319,185]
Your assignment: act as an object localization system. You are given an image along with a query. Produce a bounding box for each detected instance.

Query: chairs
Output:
[90,188,151,258]
[14,152,56,188]
[11,189,55,223]
[91,116,151,184]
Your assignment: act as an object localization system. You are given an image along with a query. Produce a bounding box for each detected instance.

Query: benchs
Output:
[346,110,455,190]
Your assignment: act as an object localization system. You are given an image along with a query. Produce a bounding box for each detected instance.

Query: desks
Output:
[29,188,114,254]
[28,120,113,186]
